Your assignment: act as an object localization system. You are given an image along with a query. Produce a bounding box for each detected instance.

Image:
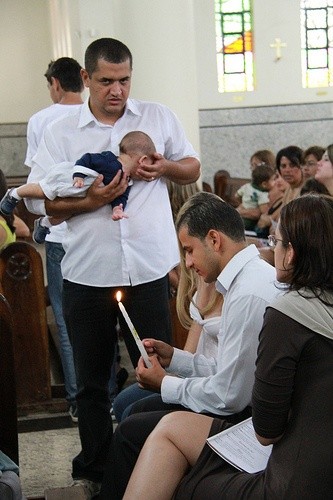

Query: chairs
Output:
[1,242,68,413]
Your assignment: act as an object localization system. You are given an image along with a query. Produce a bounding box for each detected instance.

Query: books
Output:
[205,416,273,474]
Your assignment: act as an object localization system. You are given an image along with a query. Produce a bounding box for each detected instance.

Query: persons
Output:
[234,165,276,232]
[161,175,202,299]
[113,191,225,426]
[122,194,333,500]
[0,130,156,245]
[232,143,333,267]
[99,197,290,500]
[23,38,201,500]
[0,169,30,251]
[24,57,120,427]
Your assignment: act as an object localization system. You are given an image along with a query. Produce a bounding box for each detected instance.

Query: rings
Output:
[152,177,154,181]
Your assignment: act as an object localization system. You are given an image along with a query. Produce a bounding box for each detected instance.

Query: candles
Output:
[115,290,153,368]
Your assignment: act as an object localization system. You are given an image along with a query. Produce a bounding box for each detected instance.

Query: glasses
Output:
[268,234,287,246]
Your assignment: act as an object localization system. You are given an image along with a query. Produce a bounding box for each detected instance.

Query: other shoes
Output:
[32,217,50,245]
[0,187,21,216]
